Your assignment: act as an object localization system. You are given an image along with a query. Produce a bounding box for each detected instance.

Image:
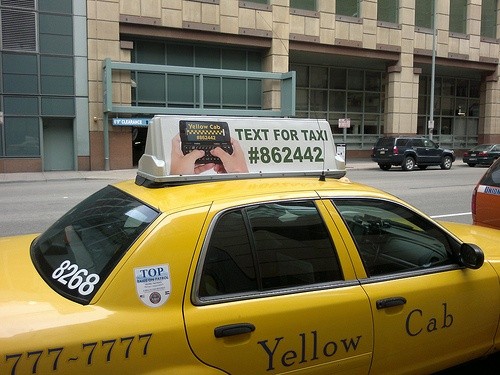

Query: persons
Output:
[170,133,248,175]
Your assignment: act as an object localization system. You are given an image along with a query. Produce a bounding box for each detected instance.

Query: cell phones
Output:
[179,120,234,164]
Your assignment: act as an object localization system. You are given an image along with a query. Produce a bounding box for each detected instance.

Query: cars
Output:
[0,114,500,375]
[463,143,500,167]
[472,156,500,230]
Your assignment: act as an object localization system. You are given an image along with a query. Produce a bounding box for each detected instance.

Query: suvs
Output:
[371,135,456,170]
